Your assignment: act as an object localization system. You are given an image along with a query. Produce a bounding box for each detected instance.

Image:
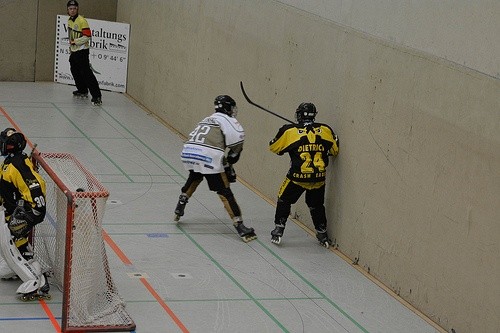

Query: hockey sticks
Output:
[239,81,298,125]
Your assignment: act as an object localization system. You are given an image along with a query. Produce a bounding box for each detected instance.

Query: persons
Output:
[269,102,339,242]
[58,0,102,102]
[0,128,51,295]
[174,94,255,237]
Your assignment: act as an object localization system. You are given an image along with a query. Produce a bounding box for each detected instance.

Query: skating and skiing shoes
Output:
[91,96,103,107]
[269,225,285,245]
[316,230,330,249]
[173,200,186,221]
[234,222,257,242]
[17,282,53,302]
[72,89,89,99]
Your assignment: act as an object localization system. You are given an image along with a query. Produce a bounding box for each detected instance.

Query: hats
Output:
[67,0,78,6]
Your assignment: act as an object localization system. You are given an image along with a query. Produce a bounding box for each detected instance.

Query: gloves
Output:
[226,148,243,164]
[226,166,237,183]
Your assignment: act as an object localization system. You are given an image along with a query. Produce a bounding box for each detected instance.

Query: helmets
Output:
[214,95,239,117]
[295,102,317,122]
[0,127,26,155]
